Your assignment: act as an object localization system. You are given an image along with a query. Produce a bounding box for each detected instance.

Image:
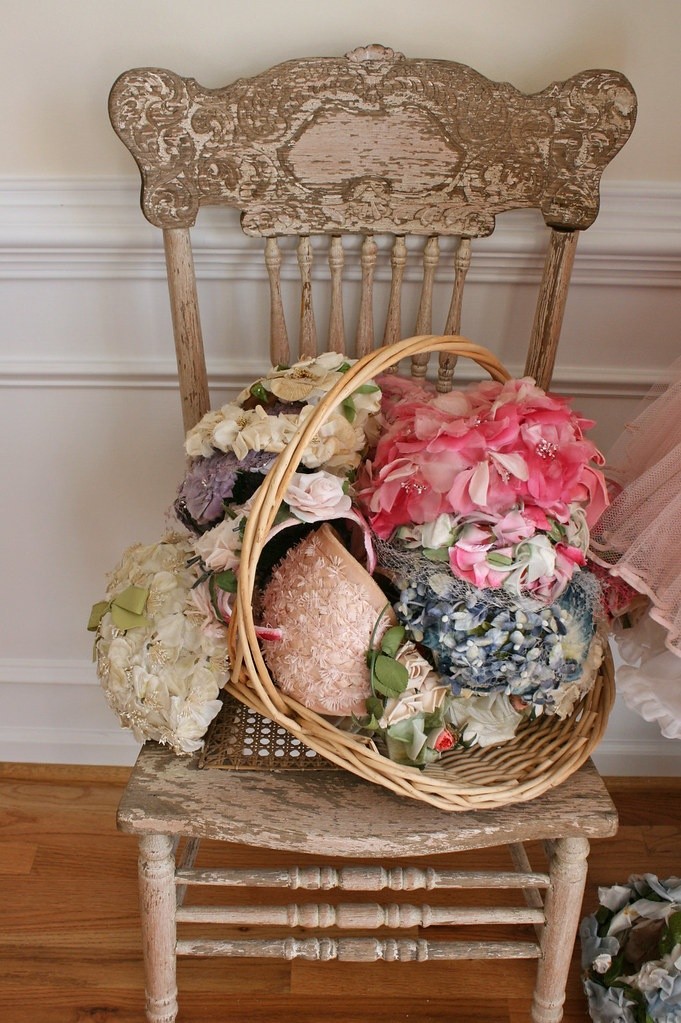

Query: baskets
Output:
[221,332,617,810]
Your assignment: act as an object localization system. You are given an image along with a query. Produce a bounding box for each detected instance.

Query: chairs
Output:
[107,44,638,1023]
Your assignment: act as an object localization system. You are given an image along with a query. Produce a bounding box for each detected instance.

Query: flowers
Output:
[91,351,607,765]
[580,873,681,1023]
